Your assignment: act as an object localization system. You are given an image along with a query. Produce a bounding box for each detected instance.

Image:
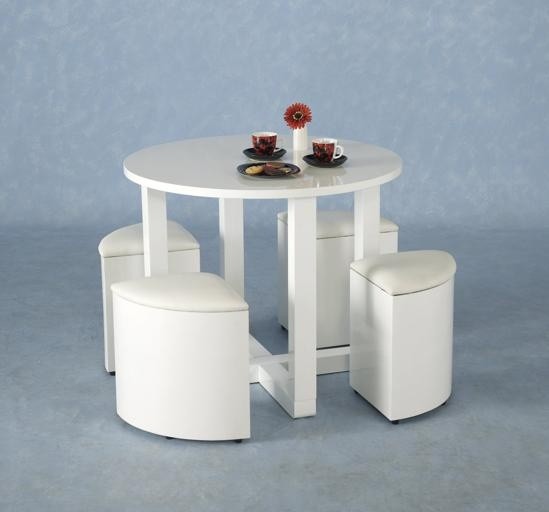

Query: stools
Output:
[101,266,252,447]
[271,206,400,353]
[94,215,203,378]
[344,246,463,424]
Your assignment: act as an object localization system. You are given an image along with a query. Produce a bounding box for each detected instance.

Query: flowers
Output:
[283,97,314,135]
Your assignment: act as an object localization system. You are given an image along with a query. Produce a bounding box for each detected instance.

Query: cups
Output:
[311,138,343,162]
[250,132,278,155]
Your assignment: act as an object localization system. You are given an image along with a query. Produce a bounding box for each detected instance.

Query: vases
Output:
[290,124,311,170]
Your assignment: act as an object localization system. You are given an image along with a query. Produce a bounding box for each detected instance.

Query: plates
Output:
[236,162,301,179]
[242,147,288,161]
[302,153,348,168]
